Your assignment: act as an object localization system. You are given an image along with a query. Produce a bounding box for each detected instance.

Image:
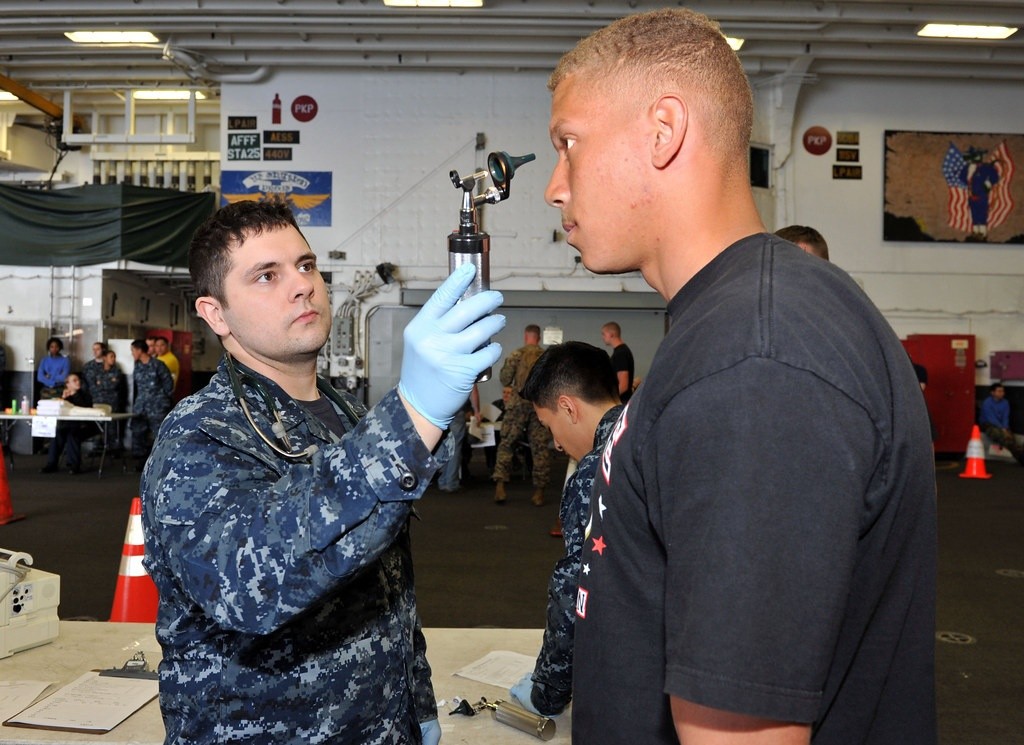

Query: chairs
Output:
[69,403,115,476]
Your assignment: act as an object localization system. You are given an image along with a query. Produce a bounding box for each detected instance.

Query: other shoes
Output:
[36,462,58,473]
[512,457,522,471]
[68,463,82,474]
[121,465,139,475]
[444,479,460,492]
[488,468,497,482]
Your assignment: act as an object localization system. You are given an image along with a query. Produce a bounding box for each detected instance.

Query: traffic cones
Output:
[108,496,159,623]
[959,424,992,478]
[0,442,27,525]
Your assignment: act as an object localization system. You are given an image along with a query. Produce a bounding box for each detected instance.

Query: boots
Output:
[529,487,545,506]
[494,478,507,501]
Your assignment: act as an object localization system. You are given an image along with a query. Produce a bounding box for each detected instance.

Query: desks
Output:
[0,413,135,480]
[0,621,573,745]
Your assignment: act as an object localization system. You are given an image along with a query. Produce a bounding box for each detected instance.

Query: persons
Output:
[544,8,937,745]
[771,224,829,264]
[139,200,507,745]
[129,335,180,483]
[911,361,941,442]
[510,340,625,719]
[81,343,127,459]
[602,321,634,406]
[37,337,71,456]
[38,373,93,475]
[492,325,549,507]
[483,384,525,476]
[979,383,1024,466]
[437,381,483,495]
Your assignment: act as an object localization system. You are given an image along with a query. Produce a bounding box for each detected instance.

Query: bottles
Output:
[21,396,29,415]
[12,400,18,415]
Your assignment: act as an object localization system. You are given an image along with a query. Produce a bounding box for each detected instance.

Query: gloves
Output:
[511,671,559,718]
[417,718,442,745]
[398,262,505,429]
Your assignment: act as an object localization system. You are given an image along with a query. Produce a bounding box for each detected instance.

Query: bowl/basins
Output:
[94,405,112,416]
